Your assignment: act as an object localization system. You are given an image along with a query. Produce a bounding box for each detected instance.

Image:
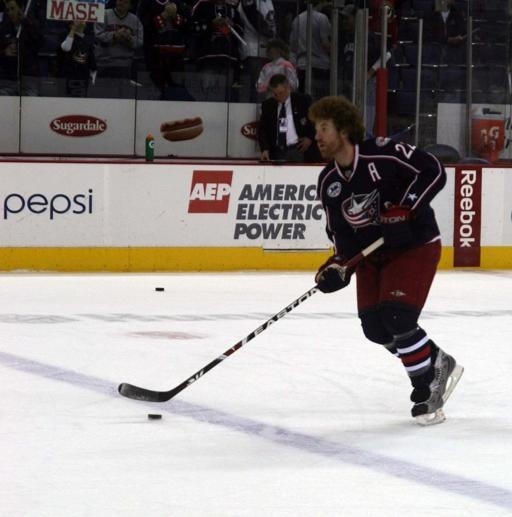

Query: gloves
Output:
[379,206,416,250]
[315,254,355,293]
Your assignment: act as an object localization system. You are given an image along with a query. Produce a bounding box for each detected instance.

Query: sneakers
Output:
[434,348,455,396]
[411,383,444,416]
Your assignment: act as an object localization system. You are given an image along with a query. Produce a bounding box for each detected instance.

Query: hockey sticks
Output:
[118,237,384,402]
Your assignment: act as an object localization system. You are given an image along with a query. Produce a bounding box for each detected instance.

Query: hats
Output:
[258,38,286,50]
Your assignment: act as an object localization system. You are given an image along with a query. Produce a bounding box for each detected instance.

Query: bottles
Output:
[144,131,155,161]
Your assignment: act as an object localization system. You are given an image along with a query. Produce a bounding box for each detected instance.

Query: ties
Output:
[276,101,287,151]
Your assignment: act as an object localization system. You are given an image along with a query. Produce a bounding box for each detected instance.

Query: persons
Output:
[255,72,320,162]
[307,93,458,422]
[0,0,511,105]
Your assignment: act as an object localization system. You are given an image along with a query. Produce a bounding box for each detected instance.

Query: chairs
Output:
[388,0,511,164]
